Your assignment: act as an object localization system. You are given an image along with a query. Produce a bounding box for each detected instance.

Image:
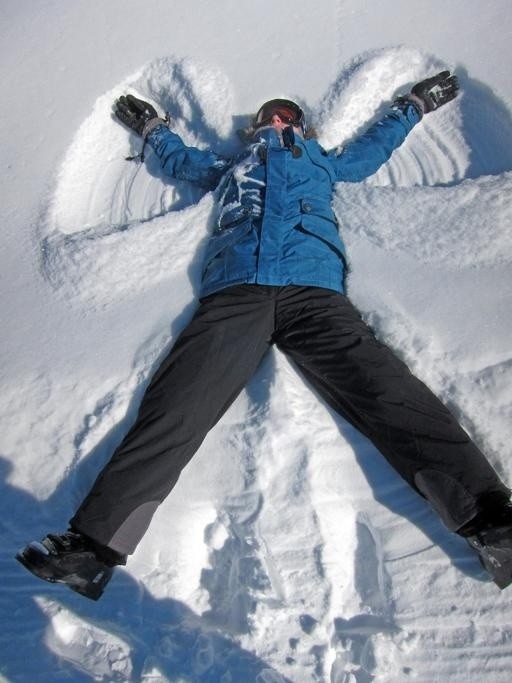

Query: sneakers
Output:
[459,491,512,590]
[14,530,126,600]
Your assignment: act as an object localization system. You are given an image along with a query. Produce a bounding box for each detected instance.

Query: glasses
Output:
[253,99,307,135]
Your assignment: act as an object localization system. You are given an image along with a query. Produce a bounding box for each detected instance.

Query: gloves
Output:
[114,95,168,140]
[394,70,461,120]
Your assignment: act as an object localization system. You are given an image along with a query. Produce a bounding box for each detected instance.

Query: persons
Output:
[12,67,512,602]
